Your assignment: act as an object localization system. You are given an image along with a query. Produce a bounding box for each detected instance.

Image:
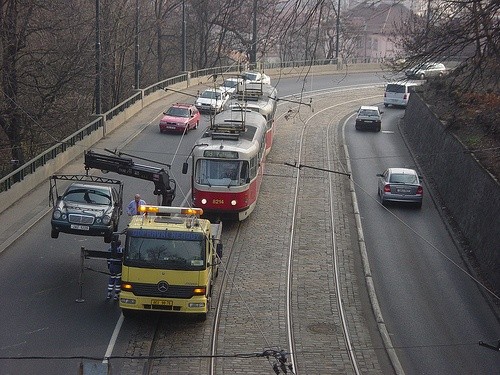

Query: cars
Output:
[196,88,230,115]
[160,103,201,134]
[355,105,383,132]
[376,167,425,207]
[50,184,121,244]
[242,73,271,85]
[218,77,245,95]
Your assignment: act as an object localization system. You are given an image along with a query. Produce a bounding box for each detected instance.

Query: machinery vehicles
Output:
[80,147,224,323]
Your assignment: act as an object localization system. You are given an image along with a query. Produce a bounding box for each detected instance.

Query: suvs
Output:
[404,62,447,79]
[384,82,418,109]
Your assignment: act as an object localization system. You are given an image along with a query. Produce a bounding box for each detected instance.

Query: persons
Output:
[107,241,125,301]
[126,194,147,217]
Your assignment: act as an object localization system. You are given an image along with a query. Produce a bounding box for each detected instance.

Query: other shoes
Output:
[114,295,117,299]
[107,294,111,298]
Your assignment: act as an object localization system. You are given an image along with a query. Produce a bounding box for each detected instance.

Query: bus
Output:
[180,74,277,223]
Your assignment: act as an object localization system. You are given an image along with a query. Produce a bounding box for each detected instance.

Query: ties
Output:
[137,202,140,214]
